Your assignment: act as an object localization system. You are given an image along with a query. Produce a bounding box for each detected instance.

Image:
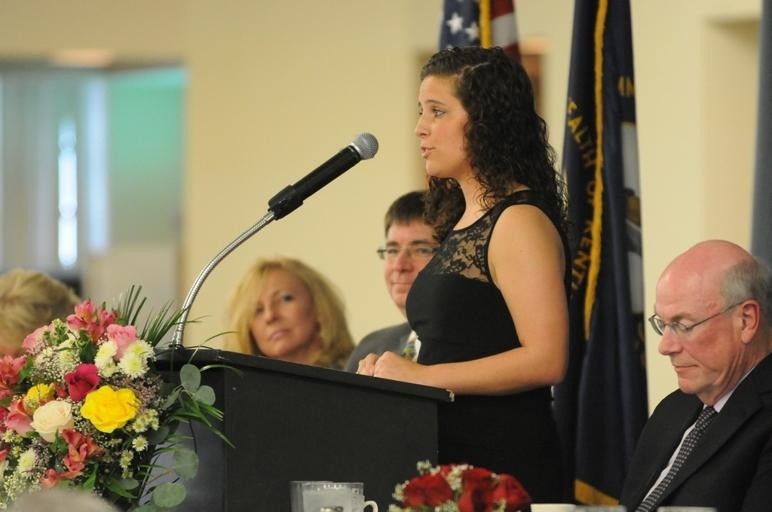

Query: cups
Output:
[284,478,380,512]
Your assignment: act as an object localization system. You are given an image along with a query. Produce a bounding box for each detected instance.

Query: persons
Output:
[340,187,443,374]
[615,238,771,512]
[359,43,582,512]
[224,256,356,373]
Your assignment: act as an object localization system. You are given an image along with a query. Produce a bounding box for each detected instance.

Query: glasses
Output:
[647,297,753,341]
[376,245,440,260]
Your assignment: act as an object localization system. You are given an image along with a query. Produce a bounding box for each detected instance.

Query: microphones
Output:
[268,132,378,219]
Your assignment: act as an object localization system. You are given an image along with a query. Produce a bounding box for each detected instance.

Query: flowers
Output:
[384,457,533,511]
